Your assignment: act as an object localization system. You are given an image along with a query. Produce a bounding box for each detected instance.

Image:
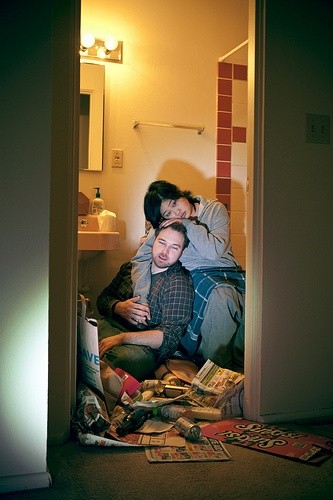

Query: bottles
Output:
[116,408,147,436]
[152,405,194,424]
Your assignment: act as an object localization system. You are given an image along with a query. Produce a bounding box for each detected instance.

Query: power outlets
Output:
[110,149,123,167]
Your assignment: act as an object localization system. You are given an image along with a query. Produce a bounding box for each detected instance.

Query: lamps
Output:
[80,33,123,62]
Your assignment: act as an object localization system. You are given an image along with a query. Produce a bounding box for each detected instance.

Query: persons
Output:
[130,180,246,368]
[96,222,195,382]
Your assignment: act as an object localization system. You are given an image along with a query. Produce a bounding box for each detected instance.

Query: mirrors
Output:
[80,63,105,172]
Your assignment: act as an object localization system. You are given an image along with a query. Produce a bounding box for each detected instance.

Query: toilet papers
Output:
[96,210,116,232]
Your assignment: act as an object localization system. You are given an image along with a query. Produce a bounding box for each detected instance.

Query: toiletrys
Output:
[90,186,105,215]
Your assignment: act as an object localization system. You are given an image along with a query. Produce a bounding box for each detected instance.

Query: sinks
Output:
[77,230,121,251]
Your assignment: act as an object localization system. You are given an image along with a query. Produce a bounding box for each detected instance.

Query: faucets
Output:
[80,219,89,228]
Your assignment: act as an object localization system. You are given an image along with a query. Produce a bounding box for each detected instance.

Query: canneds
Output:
[142,380,164,392]
[163,384,188,398]
[154,365,180,386]
[175,417,200,440]
[161,405,186,421]
